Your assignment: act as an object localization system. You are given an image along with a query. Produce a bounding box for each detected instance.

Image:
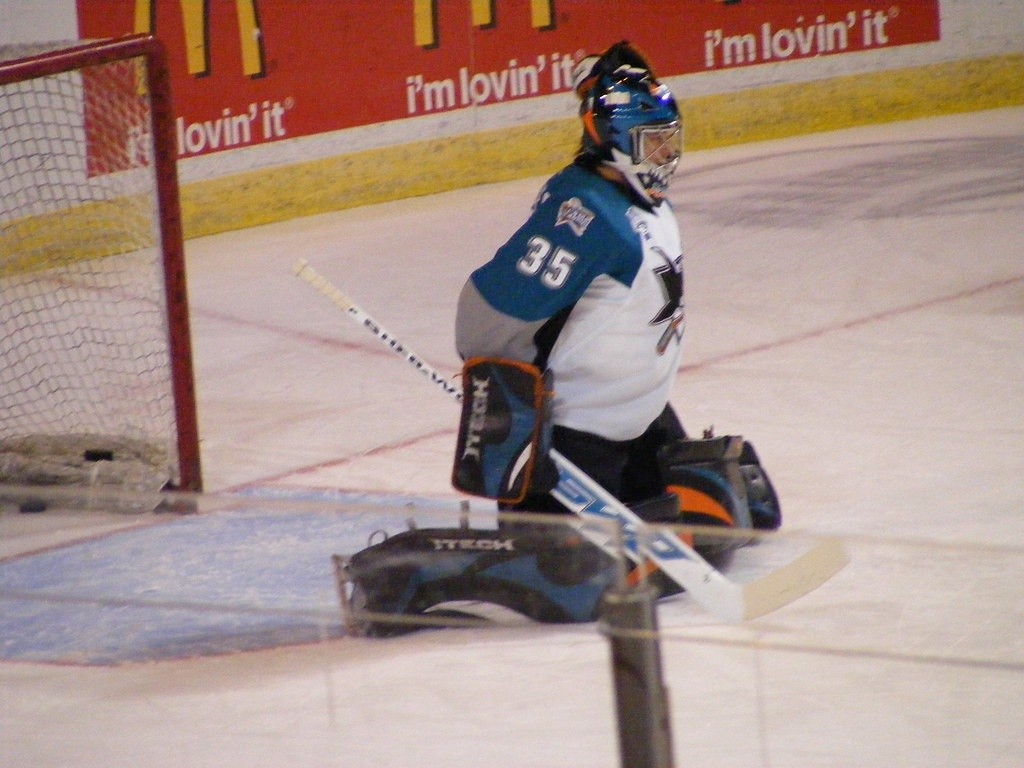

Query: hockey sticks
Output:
[290,255,852,624]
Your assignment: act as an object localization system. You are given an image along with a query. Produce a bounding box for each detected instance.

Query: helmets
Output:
[580,64,682,207]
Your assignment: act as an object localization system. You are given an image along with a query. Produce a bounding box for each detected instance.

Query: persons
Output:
[342,41,782,640]
